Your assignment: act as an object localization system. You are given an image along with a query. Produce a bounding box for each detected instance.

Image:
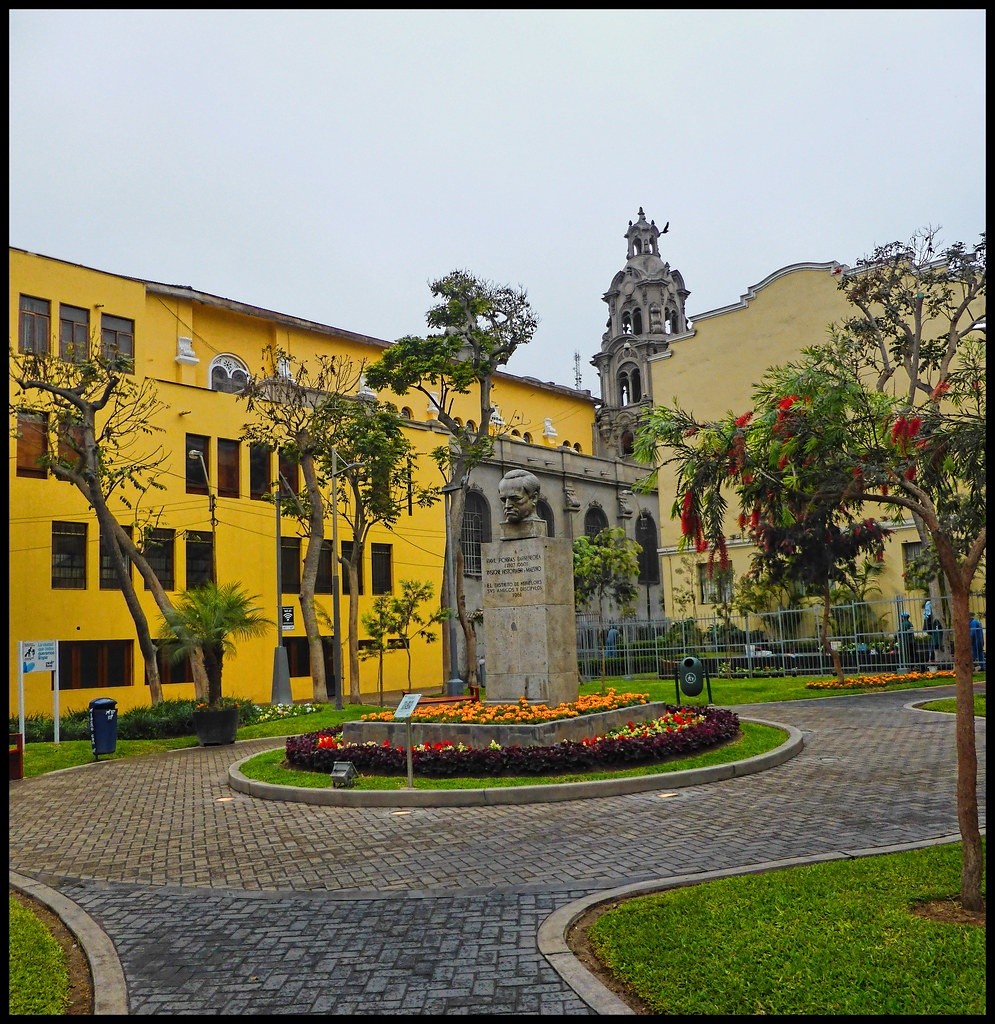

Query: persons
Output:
[596,626,621,658]
[968,612,985,671]
[892,613,917,673]
[498,469,540,521]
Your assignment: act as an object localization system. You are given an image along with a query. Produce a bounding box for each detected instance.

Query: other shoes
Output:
[978,667,986,672]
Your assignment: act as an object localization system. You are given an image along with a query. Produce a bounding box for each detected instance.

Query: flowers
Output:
[192,699,240,712]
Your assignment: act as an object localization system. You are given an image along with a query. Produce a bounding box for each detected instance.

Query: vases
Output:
[193,710,239,746]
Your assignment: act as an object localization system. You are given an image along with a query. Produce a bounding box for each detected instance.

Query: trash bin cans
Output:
[679,656,703,696]
[89,697,119,755]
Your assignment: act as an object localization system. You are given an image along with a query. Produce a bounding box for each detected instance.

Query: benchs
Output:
[402,686,481,705]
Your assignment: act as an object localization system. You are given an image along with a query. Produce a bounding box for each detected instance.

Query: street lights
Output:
[270,478,293,705]
[189,450,217,586]
[442,482,463,697]
[622,489,650,621]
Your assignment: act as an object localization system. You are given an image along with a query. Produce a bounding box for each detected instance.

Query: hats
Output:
[901,612,910,617]
[969,612,976,616]
